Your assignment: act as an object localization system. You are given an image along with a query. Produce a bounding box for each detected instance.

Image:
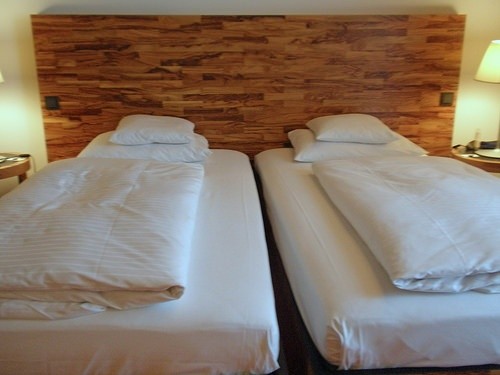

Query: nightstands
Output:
[0,154,31,185]
[452,146,500,174]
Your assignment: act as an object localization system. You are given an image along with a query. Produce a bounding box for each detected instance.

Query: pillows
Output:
[76,132,210,164]
[286,129,429,162]
[306,112,403,145]
[109,115,195,143]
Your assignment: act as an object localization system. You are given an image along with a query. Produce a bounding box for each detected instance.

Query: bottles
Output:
[473,128,481,149]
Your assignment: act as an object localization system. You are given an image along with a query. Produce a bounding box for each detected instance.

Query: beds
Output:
[0,14,499,374]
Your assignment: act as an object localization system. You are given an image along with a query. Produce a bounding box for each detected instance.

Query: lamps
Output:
[474,41,500,148]
